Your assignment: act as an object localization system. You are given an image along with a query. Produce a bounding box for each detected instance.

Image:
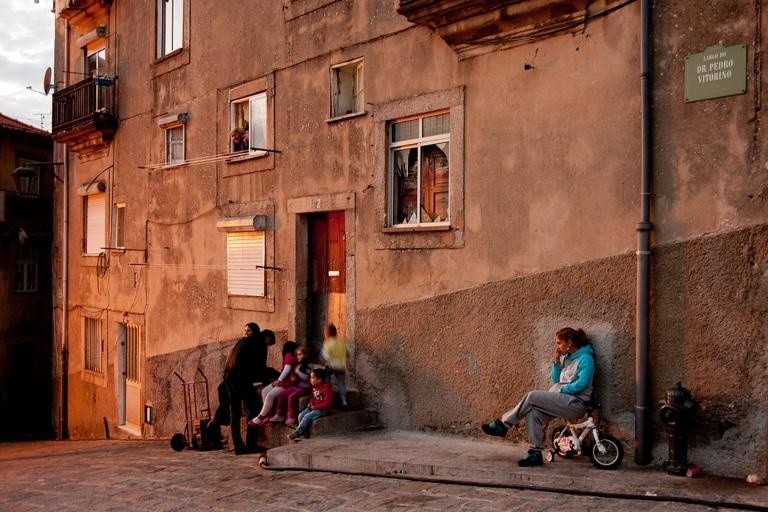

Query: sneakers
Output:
[246,414,297,427]
[482,419,508,437]
[287,431,300,440]
[235,445,268,455]
[518,448,543,467]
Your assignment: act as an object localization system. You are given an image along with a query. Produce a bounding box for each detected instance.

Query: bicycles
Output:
[544,401,625,469]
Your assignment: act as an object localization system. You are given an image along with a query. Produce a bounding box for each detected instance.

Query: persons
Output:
[245,340,298,428]
[318,322,350,407]
[230,127,242,153]
[244,128,249,136]
[286,367,335,441]
[269,345,315,428]
[240,134,249,151]
[220,329,280,455]
[480,326,596,468]
[205,320,260,431]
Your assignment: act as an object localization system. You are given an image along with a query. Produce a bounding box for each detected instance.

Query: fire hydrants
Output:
[659,382,699,477]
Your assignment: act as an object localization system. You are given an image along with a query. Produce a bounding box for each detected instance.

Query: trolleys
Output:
[169,366,213,451]
